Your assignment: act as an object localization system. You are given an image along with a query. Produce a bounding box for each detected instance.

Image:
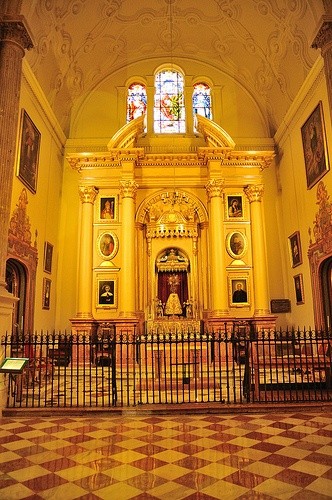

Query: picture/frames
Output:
[99,196,119,224]
[15,108,41,194]
[226,275,250,308]
[43,277,52,310]
[96,230,118,261]
[287,231,302,268]
[300,101,330,190]
[224,192,246,221]
[224,230,247,259]
[43,241,54,274]
[294,273,305,305]
[96,278,117,309]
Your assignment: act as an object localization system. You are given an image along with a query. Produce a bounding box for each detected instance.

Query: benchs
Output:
[246,341,332,401]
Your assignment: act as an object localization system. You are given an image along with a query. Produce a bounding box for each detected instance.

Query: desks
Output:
[152,318,200,336]
[145,346,207,381]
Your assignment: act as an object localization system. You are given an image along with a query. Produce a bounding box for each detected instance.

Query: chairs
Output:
[92,327,114,366]
